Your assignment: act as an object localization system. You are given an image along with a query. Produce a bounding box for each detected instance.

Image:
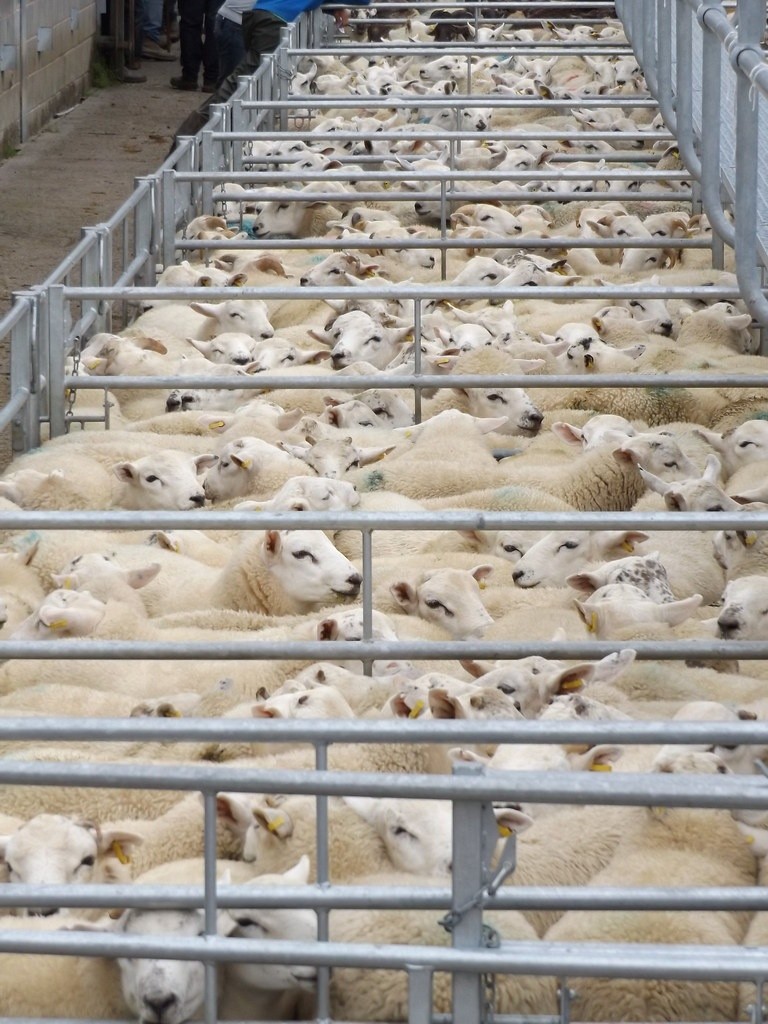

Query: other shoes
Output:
[202,84,216,93]
[169,75,198,89]
[140,35,177,60]
[119,65,147,83]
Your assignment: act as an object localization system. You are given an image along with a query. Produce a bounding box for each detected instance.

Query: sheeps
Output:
[0,0,768,1024]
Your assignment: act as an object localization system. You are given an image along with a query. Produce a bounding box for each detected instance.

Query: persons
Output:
[138,0,176,61]
[171,0,349,171]
